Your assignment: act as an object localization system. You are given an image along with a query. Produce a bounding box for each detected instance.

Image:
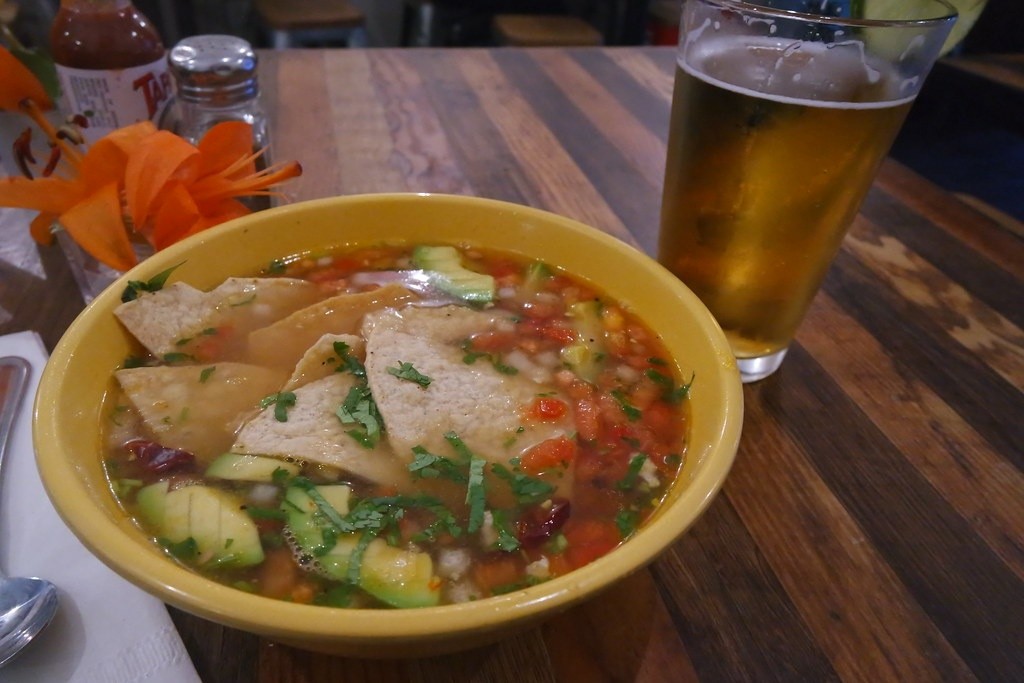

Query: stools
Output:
[252,0,370,46]
[0,44,1024,682]
[494,14,606,44]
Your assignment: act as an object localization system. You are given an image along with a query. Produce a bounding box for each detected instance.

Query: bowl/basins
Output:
[28,190,742,656]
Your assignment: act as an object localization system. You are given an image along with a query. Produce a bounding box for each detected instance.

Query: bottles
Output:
[166,34,272,214]
[50,0,177,132]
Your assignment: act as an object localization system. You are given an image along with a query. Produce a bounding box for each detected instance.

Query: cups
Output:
[657,0,957,386]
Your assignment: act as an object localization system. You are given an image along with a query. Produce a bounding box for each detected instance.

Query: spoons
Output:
[0,353,57,668]
